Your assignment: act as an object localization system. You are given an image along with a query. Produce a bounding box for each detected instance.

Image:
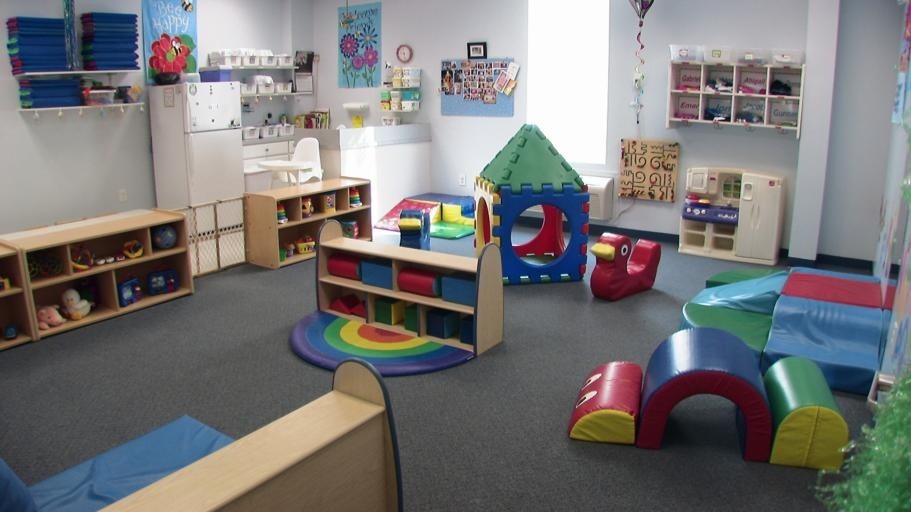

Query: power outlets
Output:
[459,176,466,185]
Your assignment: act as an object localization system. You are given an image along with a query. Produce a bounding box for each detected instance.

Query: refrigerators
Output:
[148,81,244,275]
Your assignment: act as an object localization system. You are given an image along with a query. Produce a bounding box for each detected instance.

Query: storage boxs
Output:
[243,123,294,139]
[240,74,293,94]
[208,48,294,67]
[669,44,805,68]
[198,66,233,81]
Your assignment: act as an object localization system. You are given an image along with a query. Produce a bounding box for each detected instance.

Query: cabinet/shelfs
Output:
[314,219,505,355]
[383,85,420,113]
[243,176,372,271]
[678,164,787,267]
[0,207,194,349]
[242,137,294,172]
[13,68,146,118]
[665,61,804,140]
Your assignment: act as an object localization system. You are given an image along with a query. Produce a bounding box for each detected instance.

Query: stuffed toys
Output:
[37,305,65,330]
[60,289,96,320]
[68,239,144,270]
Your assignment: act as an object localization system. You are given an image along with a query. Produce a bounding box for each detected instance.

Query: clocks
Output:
[396,44,413,62]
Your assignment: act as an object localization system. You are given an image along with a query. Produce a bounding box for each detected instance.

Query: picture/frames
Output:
[467,42,487,59]
